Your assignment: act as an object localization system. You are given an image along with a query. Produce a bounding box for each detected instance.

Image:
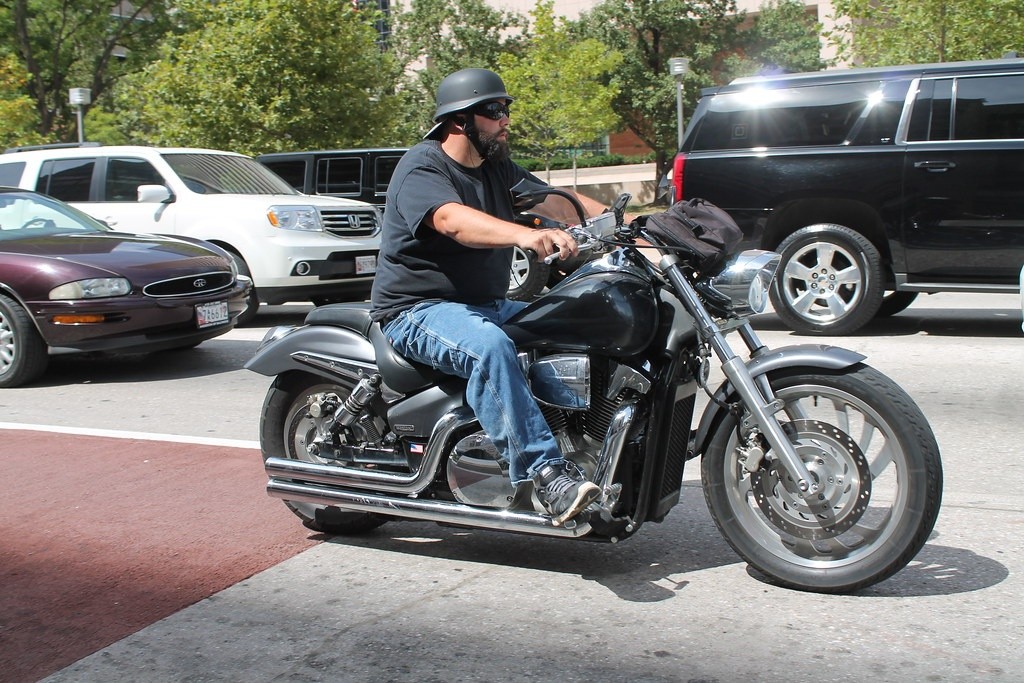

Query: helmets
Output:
[432,68,514,124]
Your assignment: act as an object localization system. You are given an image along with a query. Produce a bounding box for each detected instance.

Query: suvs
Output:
[668,57,1024,337]
[0,141,385,329]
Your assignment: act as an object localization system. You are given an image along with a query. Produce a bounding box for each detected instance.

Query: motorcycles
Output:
[244,177,943,596]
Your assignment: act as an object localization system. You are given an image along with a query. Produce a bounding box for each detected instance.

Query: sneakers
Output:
[535,464,602,527]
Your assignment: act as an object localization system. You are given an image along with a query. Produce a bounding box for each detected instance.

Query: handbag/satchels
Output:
[645,198,744,274]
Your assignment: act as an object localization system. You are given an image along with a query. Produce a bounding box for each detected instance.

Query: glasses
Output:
[470,101,510,120]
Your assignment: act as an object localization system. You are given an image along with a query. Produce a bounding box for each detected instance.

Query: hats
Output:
[423,115,450,139]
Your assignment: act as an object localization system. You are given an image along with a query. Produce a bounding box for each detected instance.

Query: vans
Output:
[260,145,413,215]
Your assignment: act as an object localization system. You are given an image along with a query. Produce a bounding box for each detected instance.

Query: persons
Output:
[370,67,639,526]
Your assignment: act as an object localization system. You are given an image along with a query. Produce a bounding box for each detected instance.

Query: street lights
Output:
[667,49,689,150]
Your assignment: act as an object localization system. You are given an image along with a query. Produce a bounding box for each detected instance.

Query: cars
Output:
[0,185,253,389]
